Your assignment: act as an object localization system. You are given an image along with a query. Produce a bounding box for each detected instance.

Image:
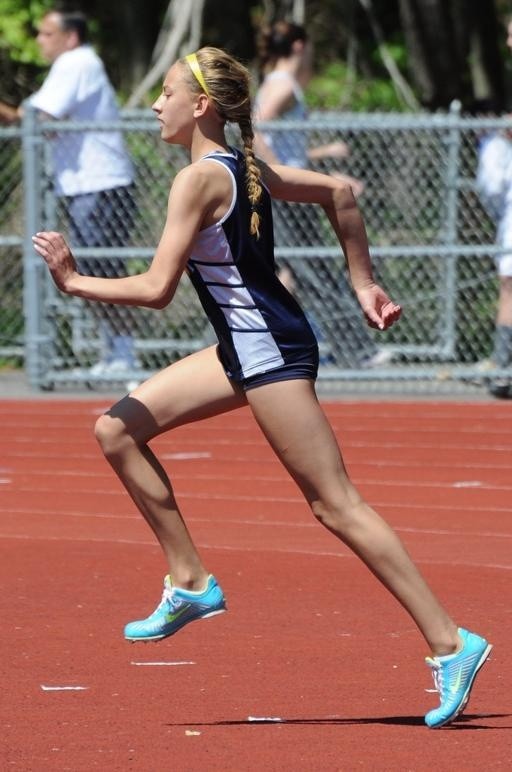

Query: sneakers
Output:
[424,624,493,729]
[123,571,227,644]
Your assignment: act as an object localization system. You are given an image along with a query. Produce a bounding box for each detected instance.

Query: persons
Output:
[18,8,145,385]
[455,106,512,403]
[30,45,498,728]
[1,60,42,211]
[247,20,393,369]
